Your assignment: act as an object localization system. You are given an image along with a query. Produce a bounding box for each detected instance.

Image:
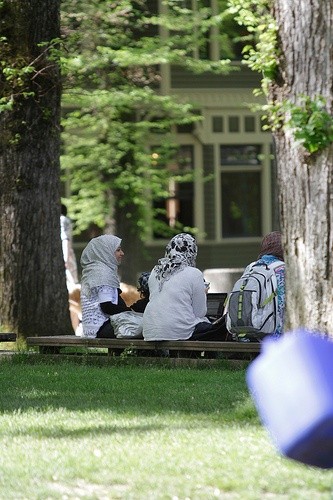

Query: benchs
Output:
[27,334,261,361]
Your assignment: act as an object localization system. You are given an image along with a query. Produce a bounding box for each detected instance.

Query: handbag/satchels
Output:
[109,308,143,338]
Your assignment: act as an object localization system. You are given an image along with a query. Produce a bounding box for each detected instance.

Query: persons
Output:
[61,204,78,284]
[80,234,135,338]
[142,233,211,342]
[237,231,285,340]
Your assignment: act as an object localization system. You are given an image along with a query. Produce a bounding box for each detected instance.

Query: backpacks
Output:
[225,261,285,337]
